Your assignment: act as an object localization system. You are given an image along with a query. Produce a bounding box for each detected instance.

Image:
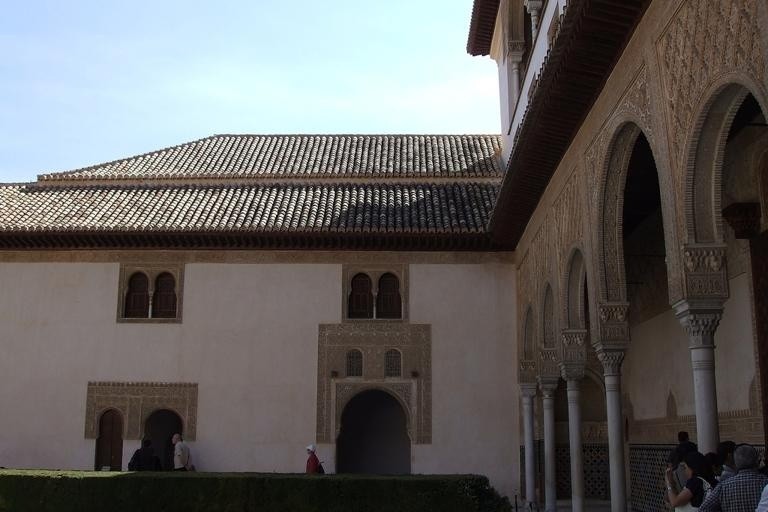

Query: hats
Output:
[307,445,316,452]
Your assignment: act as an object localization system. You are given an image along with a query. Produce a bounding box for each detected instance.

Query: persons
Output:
[305,444,324,475]
[170,433,191,471]
[663,431,767,512]
[138,438,156,472]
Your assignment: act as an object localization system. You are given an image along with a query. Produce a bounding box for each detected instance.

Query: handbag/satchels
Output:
[674,485,712,511]
